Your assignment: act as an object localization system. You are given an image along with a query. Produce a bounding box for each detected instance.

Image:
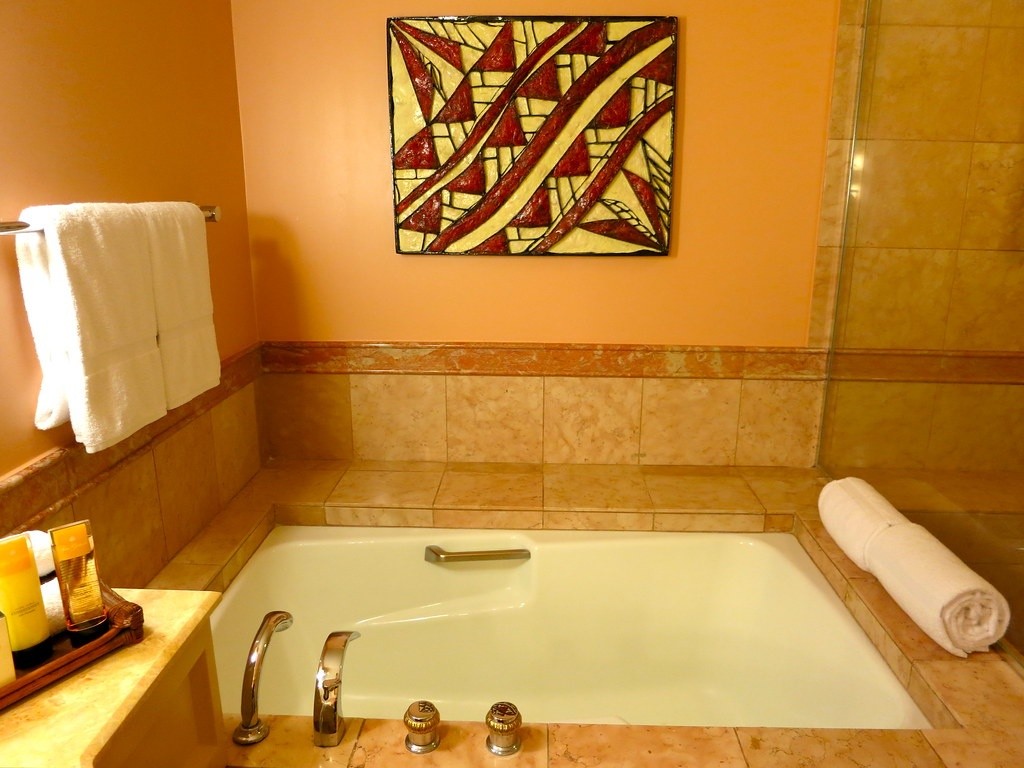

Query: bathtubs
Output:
[210,523,935,732]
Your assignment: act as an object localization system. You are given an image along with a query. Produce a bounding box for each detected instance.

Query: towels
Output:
[817,476,1011,660]
[14,200,222,455]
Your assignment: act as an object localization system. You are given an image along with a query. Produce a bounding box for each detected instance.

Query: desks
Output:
[0,587,224,768]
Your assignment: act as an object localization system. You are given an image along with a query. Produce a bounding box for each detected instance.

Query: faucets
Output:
[233,610,294,747]
[311,630,362,747]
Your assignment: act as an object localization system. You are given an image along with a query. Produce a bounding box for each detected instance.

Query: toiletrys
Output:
[0,518,111,692]
